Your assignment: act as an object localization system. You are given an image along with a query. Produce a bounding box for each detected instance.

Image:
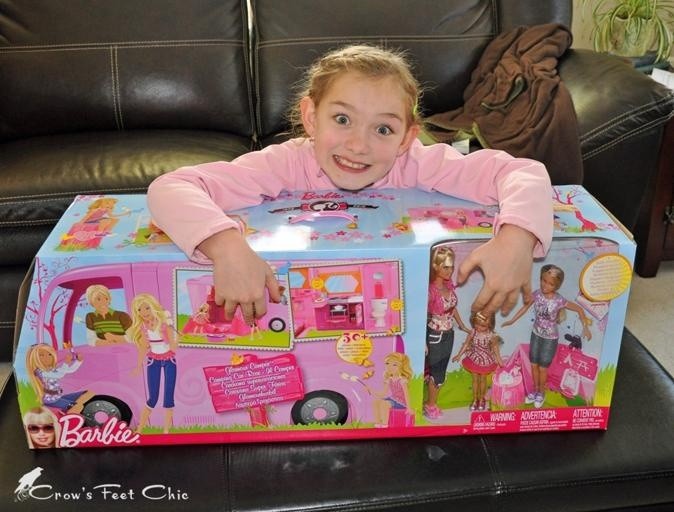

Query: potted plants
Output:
[584,0,674,56]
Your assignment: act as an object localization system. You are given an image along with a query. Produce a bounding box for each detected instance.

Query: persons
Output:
[11,181,639,449]
[141,39,557,331]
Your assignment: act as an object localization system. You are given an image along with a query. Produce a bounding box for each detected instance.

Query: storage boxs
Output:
[12,193,636,451]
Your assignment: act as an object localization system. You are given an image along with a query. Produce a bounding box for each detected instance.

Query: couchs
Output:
[0,0,673,365]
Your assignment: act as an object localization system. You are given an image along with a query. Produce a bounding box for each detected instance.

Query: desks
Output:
[0,326,673,510]
[611,50,672,260]
